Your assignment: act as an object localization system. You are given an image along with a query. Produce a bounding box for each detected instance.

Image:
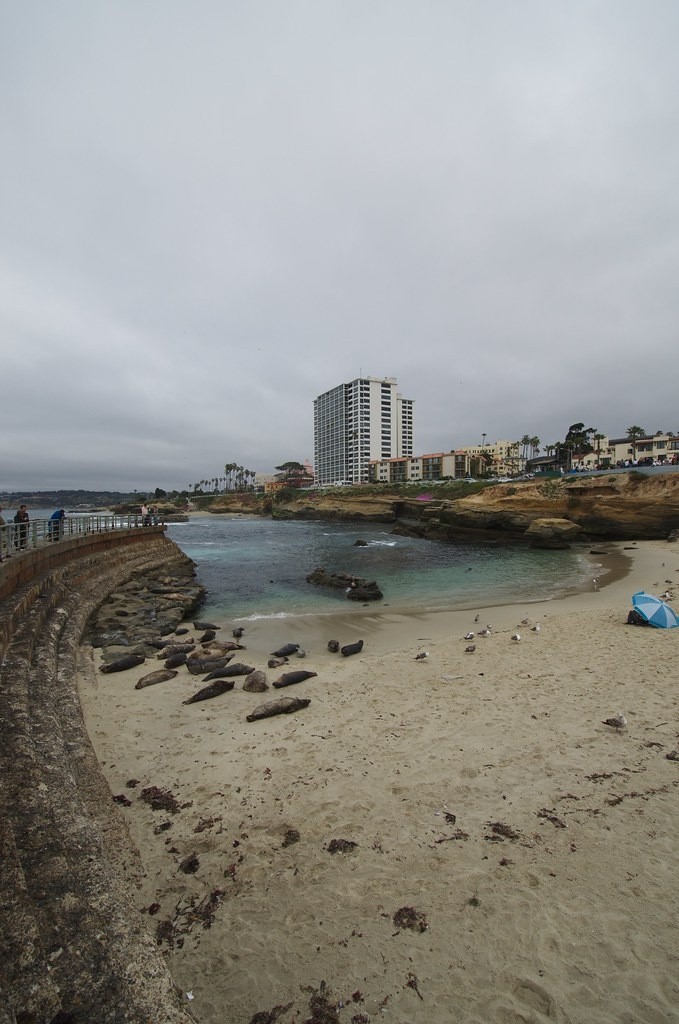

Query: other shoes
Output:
[20,549,26,551]
[149,524,152,526]
[143,525,148,527]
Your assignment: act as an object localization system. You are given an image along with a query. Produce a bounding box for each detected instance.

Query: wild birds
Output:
[592,578,600,584]
[480,623,492,636]
[530,623,541,635]
[601,712,626,732]
[463,632,475,640]
[414,651,430,662]
[474,614,480,623]
[463,644,477,653]
[510,634,521,641]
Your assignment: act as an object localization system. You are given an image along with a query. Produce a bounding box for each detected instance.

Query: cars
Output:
[464,477,514,484]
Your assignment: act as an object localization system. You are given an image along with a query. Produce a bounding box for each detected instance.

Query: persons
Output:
[48,510,67,542]
[141,502,158,526]
[626,610,649,628]
[0,505,6,547]
[540,455,679,473]
[14,504,31,552]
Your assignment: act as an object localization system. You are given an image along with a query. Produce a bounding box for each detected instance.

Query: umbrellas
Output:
[633,591,679,629]
